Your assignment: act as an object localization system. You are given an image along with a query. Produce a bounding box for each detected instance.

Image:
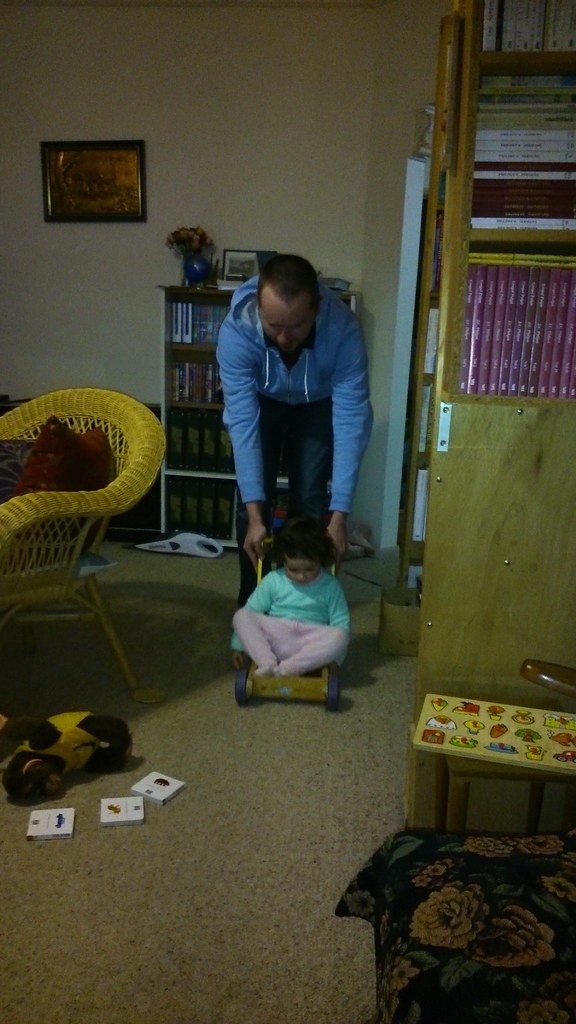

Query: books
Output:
[130,772,187,805]
[418,377,431,452]
[165,301,231,539]
[458,0,576,400]
[100,796,145,826]
[408,557,423,589]
[423,308,439,374]
[26,808,75,840]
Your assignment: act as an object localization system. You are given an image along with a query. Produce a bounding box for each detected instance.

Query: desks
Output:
[412,691,576,833]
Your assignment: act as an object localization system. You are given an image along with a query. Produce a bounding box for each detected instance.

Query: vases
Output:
[184,249,211,282]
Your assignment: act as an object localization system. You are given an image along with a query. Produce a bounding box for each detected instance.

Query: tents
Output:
[412,458,428,543]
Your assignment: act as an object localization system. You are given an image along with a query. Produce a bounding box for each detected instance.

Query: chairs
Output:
[332,802,576,1024]
[0,388,171,703]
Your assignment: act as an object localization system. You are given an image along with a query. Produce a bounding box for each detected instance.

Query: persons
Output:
[229,515,353,676]
[216,252,374,608]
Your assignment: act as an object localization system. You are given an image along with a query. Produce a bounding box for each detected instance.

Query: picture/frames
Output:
[222,249,260,282]
[39,139,147,223]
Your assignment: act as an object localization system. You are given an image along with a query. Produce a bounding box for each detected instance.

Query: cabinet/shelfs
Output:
[386,1,575,591]
[155,283,364,551]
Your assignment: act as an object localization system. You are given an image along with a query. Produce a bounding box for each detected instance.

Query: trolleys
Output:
[236,537,344,713]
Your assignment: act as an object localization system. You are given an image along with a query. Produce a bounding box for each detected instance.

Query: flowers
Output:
[165,226,214,255]
